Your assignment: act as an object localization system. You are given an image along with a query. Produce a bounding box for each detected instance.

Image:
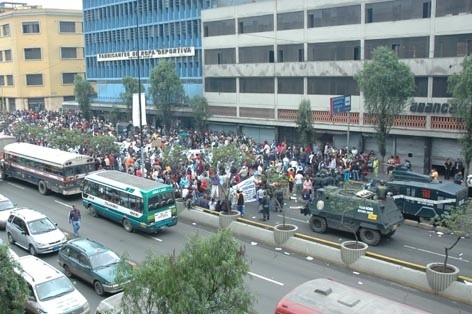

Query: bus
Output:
[80,170,178,235]
[275,265,431,314]
[0,142,94,195]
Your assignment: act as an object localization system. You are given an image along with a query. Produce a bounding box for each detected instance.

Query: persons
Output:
[467,173,472,198]
[68,204,81,237]
[431,168,438,179]
[454,158,463,175]
[0,109,412,222]
[444,158,452,180]
[454,171,463,185]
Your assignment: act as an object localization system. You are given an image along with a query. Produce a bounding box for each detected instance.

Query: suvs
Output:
[362,166,468,225]
[9,255,90,314]
[300,185,404,245]
[95,287,173,314]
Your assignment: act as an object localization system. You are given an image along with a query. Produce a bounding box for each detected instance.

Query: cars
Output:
[6,208,67,256]
[0,194,14,230]
[57,238,138,295]
